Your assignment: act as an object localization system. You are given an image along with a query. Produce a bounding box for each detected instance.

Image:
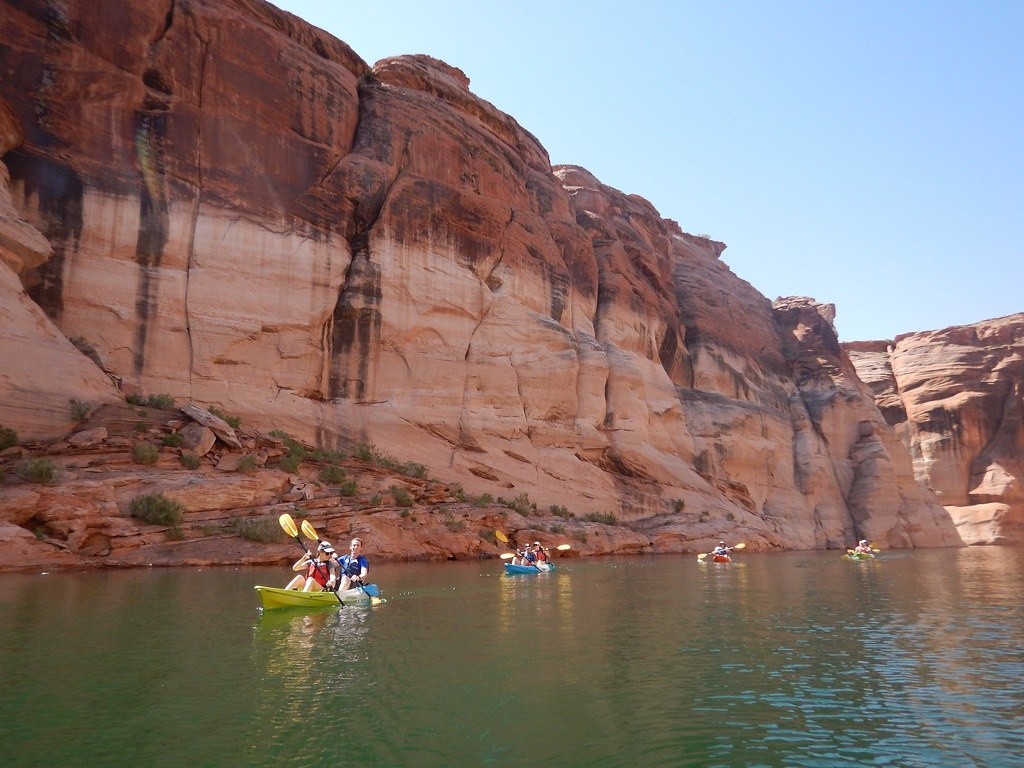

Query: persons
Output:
[284,538,371,593]
[856,540,871,554]
[714,541,729,558]
[512,542,551,566]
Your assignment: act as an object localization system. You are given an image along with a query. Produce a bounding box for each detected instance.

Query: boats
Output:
[504,563,557,576]
[840,550,876,561]
[253,583,381,611]
[712,553,732,563]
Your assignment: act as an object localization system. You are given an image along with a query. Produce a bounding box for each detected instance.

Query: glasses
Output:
[533,544,537,546]
[322,549,333,555]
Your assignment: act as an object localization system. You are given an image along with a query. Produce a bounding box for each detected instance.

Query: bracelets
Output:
[358,576,362,580]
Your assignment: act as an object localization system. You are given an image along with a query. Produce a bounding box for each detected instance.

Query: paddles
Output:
[302,519,374,599]
[500,544,571,559]
[496,530,542,573]
[847,548,881,554]
[697,543,746,558]
[847,542,878,557]
[279,512,344,604]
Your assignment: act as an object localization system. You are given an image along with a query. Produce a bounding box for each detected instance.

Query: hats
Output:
[720,541,724,544]
[715,546,722,551]
[524,544,531,550]
[533,541,541,546]
[859,541,863,544]
[862,540,867,542]
[317,541,335,552]
